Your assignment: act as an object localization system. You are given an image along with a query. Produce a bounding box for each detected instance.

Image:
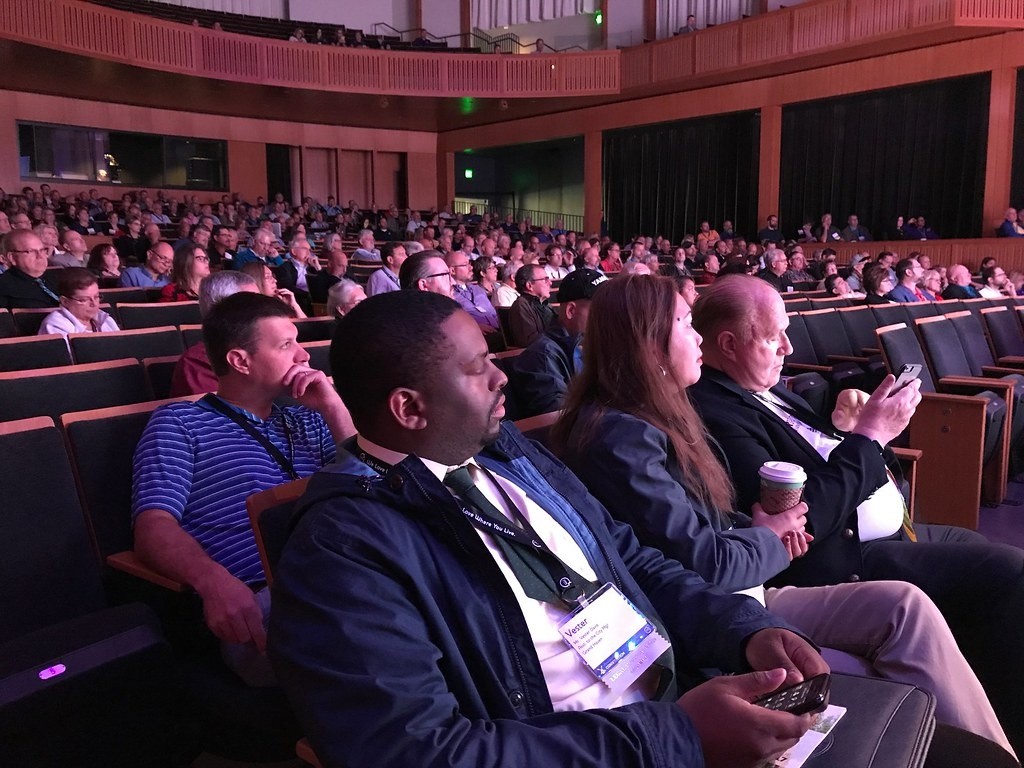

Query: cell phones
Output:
[888,363,923,398]
[749,673,832,716]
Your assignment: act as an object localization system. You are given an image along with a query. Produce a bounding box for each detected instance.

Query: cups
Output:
[758,461,807,515]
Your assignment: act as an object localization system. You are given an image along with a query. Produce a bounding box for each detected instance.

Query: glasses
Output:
[296,246,312,252]
[487,266,497,271]
[881,279,892,282]
[451,262,472,268]
[15,221,32,224]
[532,277,549,283]
[334,239,342,242]
[67,295,104,304]
[10,247,49,255]
[341,300,362,305]
[265,273,275,281]
[550,253,562,256]
[194,255,210,263]
[216,233,232,238]
[132,222,143,226]
[150,249,172,265]
[426,271,452,282]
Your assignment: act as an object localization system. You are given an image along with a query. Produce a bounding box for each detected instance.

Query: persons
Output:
[679,15,697,34]
[171,271,260,398]
[132,291,359,654]
[273,292,1022,768]
[512,269,608,419]
[190,18,543,55]
[0,182,1024,367]
[685,272,1024,768]
[549,273,1020,768]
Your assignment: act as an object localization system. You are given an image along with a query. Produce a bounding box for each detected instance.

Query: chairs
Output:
[0,203,1024,768]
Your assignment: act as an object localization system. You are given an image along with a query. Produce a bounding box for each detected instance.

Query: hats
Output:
[556,269,607,303]
[849,253,871,270]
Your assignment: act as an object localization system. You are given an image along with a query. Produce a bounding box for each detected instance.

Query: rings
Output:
[305,371,308,376]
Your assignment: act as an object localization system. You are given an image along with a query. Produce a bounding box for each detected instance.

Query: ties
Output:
[34,278,61,302]
[756,390,918,543]
[443,466,675,702]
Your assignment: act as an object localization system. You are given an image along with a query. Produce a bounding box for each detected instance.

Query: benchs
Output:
[88,0,481,53]
